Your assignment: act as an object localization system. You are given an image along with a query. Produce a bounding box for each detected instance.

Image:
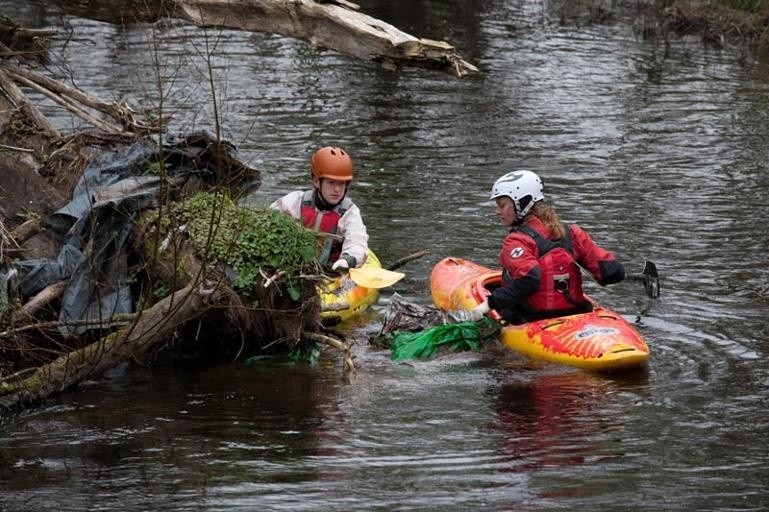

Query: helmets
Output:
[309,145,354,182]
[488,169,546,203]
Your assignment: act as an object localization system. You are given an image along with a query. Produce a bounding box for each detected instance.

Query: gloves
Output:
[472,299,492,322]
[331,257,350,273]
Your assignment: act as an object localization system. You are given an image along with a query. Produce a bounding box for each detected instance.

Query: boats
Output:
[428,256,651,369]
[318,246,382,322]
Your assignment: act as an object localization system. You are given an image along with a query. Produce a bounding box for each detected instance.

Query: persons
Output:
[473,168,628,318]
[260,144,369,276]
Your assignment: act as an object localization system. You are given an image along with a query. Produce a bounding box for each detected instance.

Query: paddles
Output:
[581,262,660,298]
[320,264,405,288]
